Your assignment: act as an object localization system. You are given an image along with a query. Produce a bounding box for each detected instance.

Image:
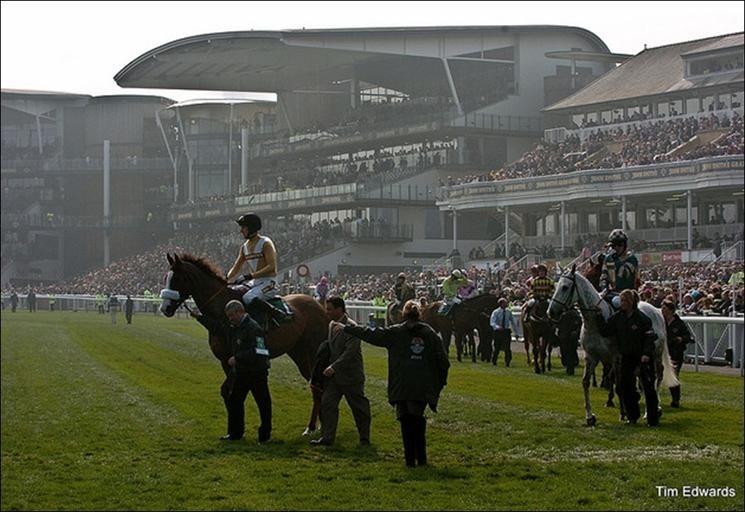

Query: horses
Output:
[388,279,502,365]
[520,292,554,374]
[159,249,332,445]
[585,258,642,408]
[547,263,683,427]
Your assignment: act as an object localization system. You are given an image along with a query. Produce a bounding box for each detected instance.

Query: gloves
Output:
[234,273,253,285]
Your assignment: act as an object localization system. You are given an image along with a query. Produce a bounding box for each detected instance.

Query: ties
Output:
[502,311,505,328]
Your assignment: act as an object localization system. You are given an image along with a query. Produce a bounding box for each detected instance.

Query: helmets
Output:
[608,230,628,241]
[531,264,548,271]
[452,269,461,277]
[236,214,262,234]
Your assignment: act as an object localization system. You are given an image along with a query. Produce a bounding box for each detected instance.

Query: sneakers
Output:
[310,438,330,445]
[219,434,235,441]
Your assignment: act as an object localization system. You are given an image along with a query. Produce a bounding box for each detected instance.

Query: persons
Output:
[1,95,743,473]
